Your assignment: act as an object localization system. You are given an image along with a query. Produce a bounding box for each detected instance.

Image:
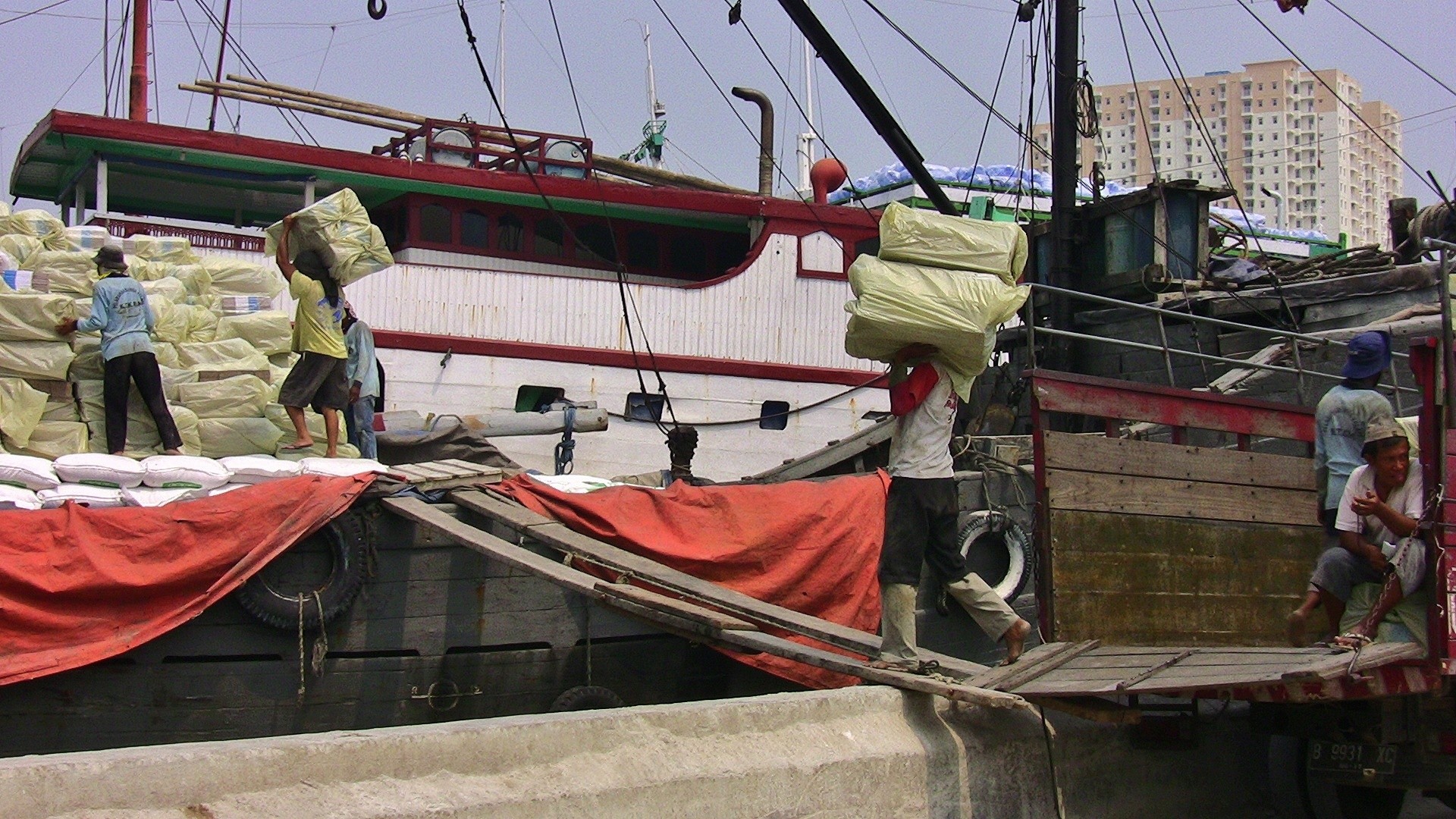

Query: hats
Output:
[1360,415,1408,458]
[1342,330,1391,378]
[91,245,127,268]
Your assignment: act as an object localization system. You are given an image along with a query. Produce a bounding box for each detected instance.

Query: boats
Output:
[1,0,1456,759]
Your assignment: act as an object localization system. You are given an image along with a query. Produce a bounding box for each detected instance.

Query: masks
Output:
[97,266,109,276]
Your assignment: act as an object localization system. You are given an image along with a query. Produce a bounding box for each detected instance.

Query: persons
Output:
[339,300,381,460]
[1286,420,1426,653]
[54,244,185,457]
[273,214,348,460]
[863,338,1032,672]
[1312,330,1396,550]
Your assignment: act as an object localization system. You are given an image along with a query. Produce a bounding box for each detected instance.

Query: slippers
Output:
[280,444,312,452]
[1334,633,1372,649]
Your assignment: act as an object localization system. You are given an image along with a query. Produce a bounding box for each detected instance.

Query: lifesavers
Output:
[548,685,628,713]
[941,507,1034,608]
[232,505,368,632]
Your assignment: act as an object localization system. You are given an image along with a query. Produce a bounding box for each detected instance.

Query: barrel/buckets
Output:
[407,128,475,168]
[504,141,587,179]
